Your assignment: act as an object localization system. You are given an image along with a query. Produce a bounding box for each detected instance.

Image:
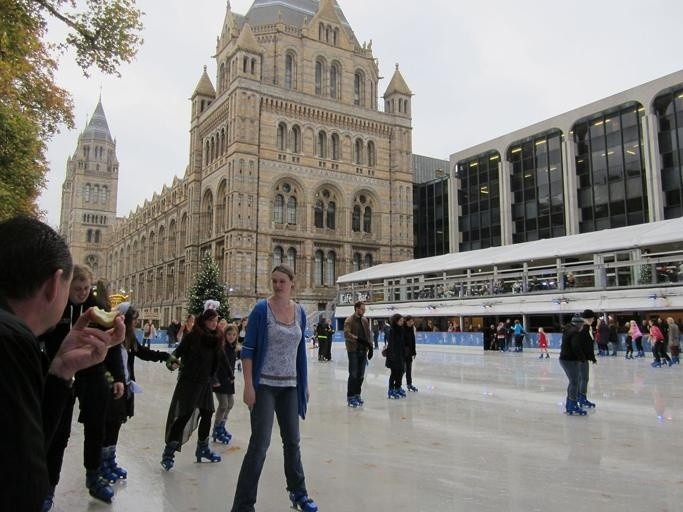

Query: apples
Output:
[90,306,121,328]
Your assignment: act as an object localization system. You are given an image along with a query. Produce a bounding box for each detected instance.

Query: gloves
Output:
[368,346,374,360]
[358,335,371,344]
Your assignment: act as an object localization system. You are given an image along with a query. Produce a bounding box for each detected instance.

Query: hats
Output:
[582,309,595,318]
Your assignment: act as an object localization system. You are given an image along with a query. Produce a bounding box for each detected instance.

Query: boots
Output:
[87,448,129,503]
[347,393,364,408]
[564,394,596,416]
[160,445,177,471]
[388,382,419,400]
[288,488,318,512]
[194,439,221,464]
[596,348,680,368]
[212,421,232,444]
[502,345,551,359]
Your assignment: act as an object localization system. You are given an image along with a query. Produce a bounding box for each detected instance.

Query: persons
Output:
[159,309,221,471]
[35,265,125,511]
[140,319,157,349]
[558,312,588,416]
[384,314,411,399]
[341,282,372,304]
[310,316,335,361]
[535,359,549,392]
[211,323,240,443]
[510,271,576,293]
[535,326,551,358]
[416,280,504,299]
[577,308,598,410]
[344,301,375,407]
[100,302,177,484]
[167,313,248,374]
[399,315,419,393]
[0,217,127,512]
[477,319,527,352]
[587,355,666,420]
[594,316,682,368]
[230,264,319,511]
[373,319,472,350]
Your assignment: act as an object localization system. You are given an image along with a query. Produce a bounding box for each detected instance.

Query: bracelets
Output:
[48,368,75,389]
